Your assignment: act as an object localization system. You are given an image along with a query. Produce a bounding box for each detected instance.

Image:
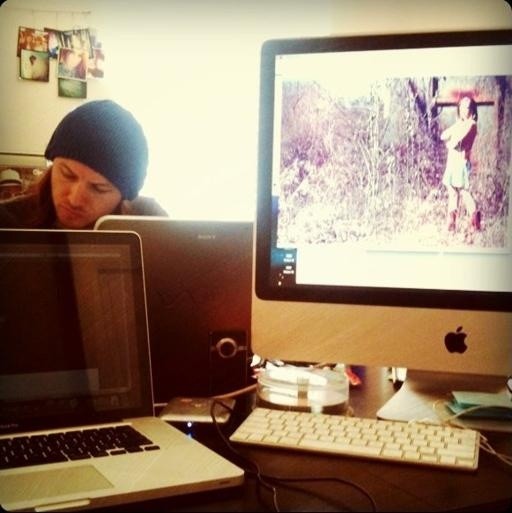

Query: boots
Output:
[470,212,480,231]
[447,209,456,232]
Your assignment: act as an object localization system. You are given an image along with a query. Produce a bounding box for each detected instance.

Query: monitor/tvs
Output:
[248,28,512,436]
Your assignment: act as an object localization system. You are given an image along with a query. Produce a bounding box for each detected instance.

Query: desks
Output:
[0,361,512,513]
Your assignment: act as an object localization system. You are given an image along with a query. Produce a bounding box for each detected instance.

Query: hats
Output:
[44,100,147,199]
[0,169,22,186]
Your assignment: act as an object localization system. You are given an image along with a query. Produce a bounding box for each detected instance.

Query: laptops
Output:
[0,229,245,513]
[92,214,313,408]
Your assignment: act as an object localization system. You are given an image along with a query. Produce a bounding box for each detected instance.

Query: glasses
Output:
[0,190,23,199]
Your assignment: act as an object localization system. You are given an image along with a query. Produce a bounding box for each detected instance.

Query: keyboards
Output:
[227,406,482,472]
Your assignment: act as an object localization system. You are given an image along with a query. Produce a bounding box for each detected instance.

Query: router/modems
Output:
[157,395,239,427]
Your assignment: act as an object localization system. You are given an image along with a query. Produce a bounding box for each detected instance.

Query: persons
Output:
[440,96,481,234]
[0,100,171,230]
